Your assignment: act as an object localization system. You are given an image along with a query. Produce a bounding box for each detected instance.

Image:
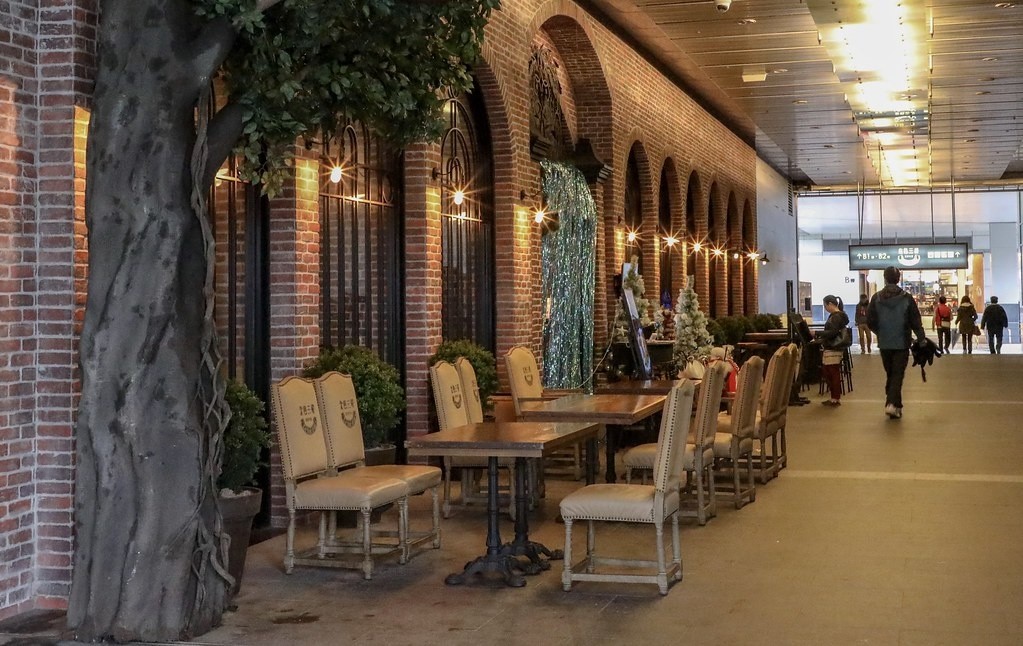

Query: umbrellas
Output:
[952,324,959,349]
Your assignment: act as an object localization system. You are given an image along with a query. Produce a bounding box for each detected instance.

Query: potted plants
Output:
[218,340,501,591]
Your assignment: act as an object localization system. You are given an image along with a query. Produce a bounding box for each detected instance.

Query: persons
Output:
[809,295,850,407]
[867,266,927,420]
[956,296,978,355]
[981,296,1008,355]
[855,294,872,355]
[932,296,953,353]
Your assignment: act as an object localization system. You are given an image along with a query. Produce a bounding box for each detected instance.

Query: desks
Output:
[745,326,824,405]
[521,394,668,522]
[595,380,702,444]
[400,422,599,587]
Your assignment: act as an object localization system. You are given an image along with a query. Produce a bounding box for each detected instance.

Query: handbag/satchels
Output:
[940,318,950,329]
[974,326,981,336]
[823,314,852,349]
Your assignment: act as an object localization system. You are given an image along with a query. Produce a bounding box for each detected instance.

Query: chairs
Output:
[268,340,800,595]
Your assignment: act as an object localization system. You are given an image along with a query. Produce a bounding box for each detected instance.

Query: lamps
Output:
[520,187,551,225]
[759,249,770,265]
[305,132,345,182]
[432,165,464,204]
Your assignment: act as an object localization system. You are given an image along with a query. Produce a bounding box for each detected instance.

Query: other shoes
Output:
[837,401,842,406]
[861,350,865,354]
[963,350,967,353]
[940,352,944,354]
[868,349,871,353]
[944,347,950,354]
[821,400,837,406]
[885,403,902,418]
